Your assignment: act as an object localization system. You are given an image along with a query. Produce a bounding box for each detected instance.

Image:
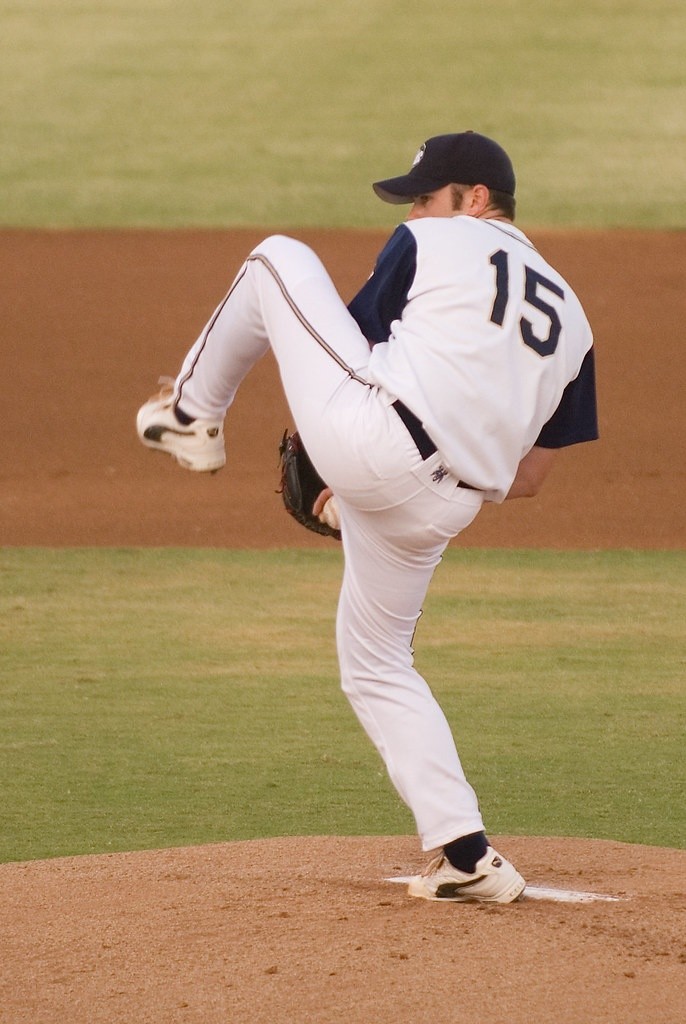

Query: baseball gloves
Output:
[275,426,345,546]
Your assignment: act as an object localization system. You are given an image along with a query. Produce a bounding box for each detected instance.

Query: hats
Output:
[371,130,516,204]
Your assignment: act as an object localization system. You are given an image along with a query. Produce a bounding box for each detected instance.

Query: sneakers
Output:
[137,400,226,472]
[407,846,526,903]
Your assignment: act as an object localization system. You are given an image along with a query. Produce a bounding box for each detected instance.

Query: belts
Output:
[392,399,486,491]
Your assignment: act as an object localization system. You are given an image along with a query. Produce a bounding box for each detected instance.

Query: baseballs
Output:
[321,492,346,532]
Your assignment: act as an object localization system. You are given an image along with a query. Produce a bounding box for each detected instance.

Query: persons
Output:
[135,130,600,906]
